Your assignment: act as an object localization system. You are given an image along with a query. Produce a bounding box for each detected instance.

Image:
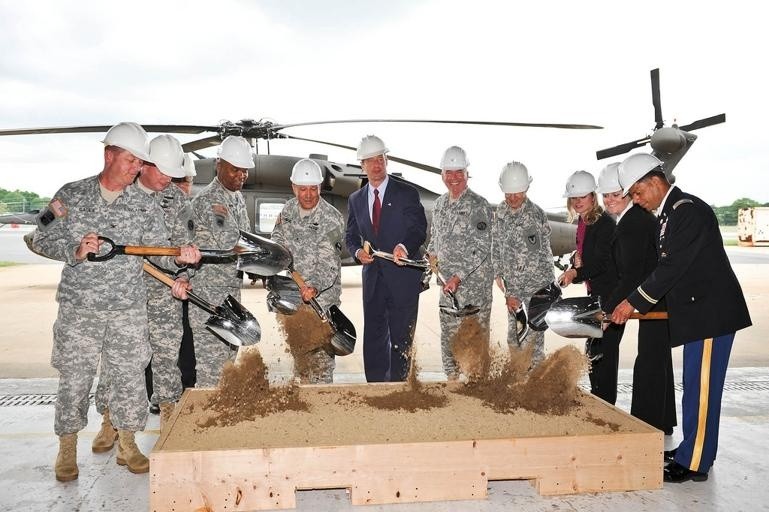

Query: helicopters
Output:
[0,67,727,288]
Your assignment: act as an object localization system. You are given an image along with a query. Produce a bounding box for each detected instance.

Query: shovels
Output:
[87,230,291,277]
[362,240,431,290]
[528,264,573,332]
[143,262,262,347]
[437,268,480,318]
[292,271,356,356]
[544,296,669,338]
[514,301,527,346]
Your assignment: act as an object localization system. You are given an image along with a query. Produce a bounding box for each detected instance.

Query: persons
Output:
[92,134,197,453]
[598,162,676,434]
[428,146,493,381]
[193,135,259,388]
[344,137,428,382]
[491,160,555,380]
[268,160,344,385]
[557,171,628,409]
[612,152,752,483]
[25,123,202,482]
[146,153,195,415]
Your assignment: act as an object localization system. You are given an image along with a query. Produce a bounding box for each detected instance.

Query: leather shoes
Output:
[149,402,159,413]
[664,447,679,463]
[664,460,708,483]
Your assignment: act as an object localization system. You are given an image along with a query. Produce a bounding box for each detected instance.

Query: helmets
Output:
[219,136,255,168]
[440,145,470,171]
[499,161,533,194]
[596,162,622,193]
[290,158,322,185]
[357,135,389,159]
[618,153,664,196]
[102,123,149,161]
[183,154,196,176]
[148,135,185,178]
[566,171,595,196]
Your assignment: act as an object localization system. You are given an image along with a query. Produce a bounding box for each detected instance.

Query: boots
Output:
[55,432,79,482]
[92,406,119,453]
[159,402,176,435]
[117,429,149,473]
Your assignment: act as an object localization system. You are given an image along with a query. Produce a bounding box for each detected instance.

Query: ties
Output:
[371,189,381,236]
